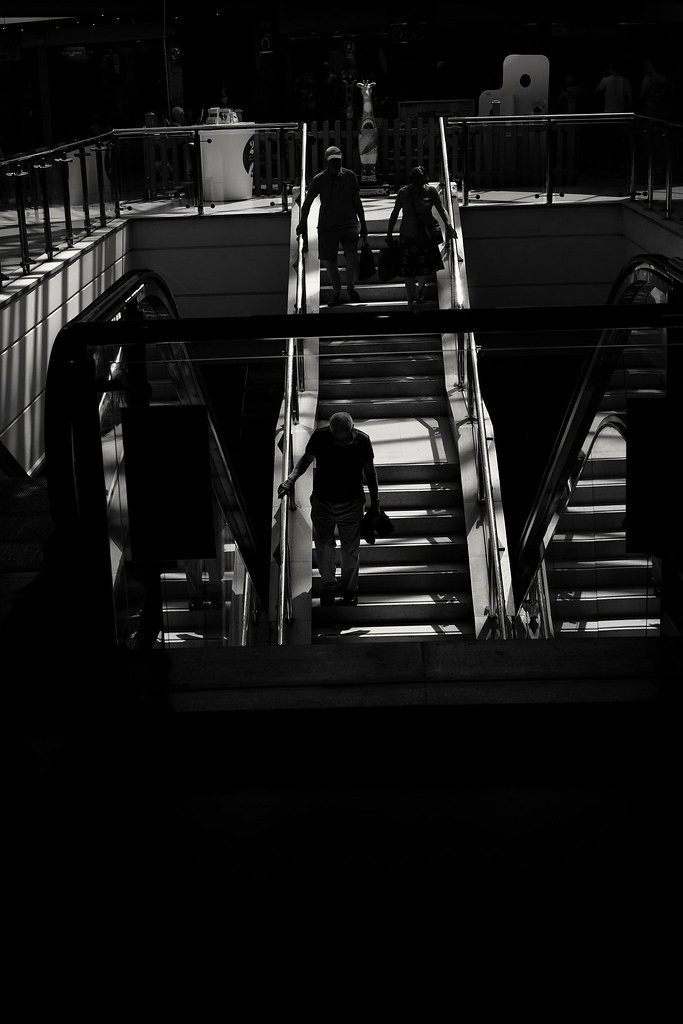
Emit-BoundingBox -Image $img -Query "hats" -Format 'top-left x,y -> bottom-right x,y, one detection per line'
325,146 -> 343,161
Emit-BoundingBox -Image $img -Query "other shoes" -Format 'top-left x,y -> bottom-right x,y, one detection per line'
188,599 -> 211,611
346,286 -> 361,302
320,597 -> 335,606
417,296 -> 425,304
342,594 -> 358,607
408,298 -> 417,311
327,296 -> 345,307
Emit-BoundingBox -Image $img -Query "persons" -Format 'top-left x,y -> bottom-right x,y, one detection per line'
638,59 -> 674,118
599,63 -> 632,112
385,167 -> 457,309
278,412 -> 378,607
170,107 -> 184,127
297,146 -> 366,307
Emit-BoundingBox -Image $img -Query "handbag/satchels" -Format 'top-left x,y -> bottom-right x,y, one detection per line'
418,218 -> 443,246
378,245 -> 399,275
360,239 -> 376,275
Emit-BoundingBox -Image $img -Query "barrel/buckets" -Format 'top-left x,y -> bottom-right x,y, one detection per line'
198,121 -> 255,201
